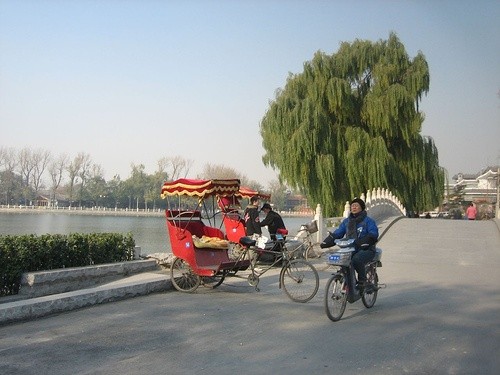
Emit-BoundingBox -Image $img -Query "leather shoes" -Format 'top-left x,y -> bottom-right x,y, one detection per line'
358,282 -> 366,288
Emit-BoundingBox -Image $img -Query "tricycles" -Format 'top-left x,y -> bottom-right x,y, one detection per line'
214,185 -> 333,271
160,177 -> 319,302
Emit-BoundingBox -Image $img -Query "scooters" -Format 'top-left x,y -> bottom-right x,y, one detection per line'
325,227 -> 387,322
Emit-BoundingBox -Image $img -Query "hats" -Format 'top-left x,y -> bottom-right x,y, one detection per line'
260,203 -> 273,210
350,198 -> 365,211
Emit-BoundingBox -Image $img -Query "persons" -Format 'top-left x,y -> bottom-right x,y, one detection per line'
408,213 -> 431,218
260,203 -> 286,267
466,204 -> 477,220
327,197 -> 379,289
244,196 -> 264,272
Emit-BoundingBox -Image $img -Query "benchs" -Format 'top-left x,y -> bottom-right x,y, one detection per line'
166,210 -> 224,249
